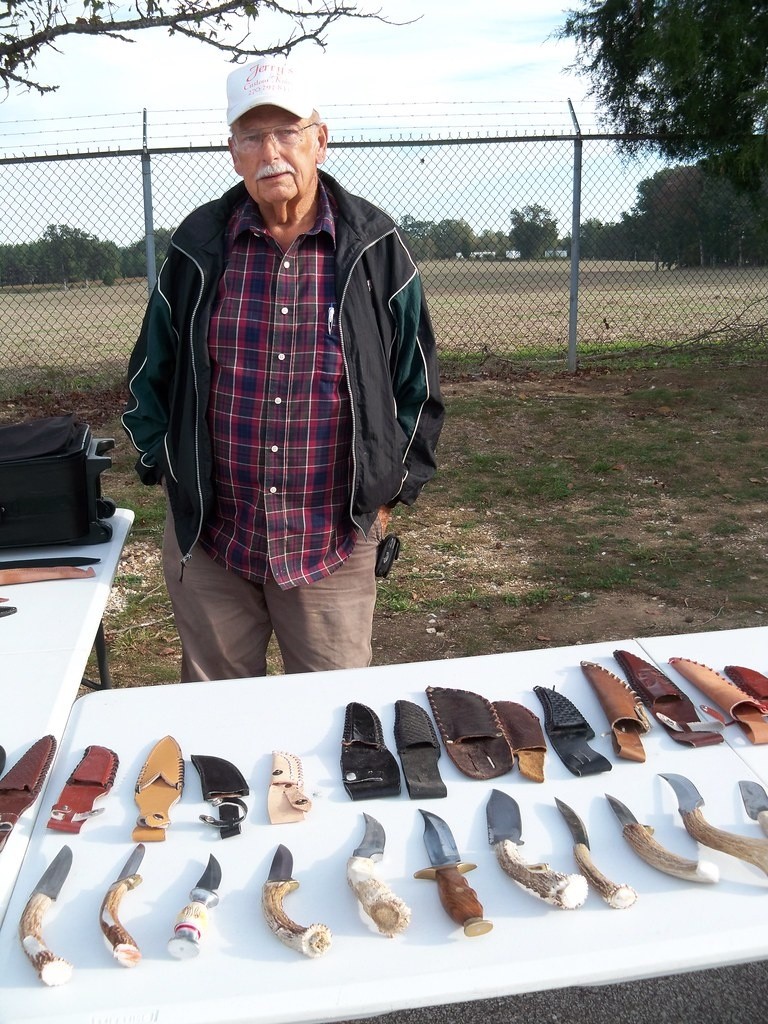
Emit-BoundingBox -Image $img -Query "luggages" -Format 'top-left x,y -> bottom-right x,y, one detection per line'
0,414 -> 116,550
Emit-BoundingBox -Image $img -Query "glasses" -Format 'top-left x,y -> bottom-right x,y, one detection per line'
233,122 -> 318,150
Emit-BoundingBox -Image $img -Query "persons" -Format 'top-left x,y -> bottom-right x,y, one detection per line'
121,58 -> 445,682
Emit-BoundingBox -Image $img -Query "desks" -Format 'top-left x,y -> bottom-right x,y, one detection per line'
0,620 -> 768,1024
0,506 -> 134,928
633,626 -> 768,794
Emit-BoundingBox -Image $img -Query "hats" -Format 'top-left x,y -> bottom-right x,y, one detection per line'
227,60 -> 317,125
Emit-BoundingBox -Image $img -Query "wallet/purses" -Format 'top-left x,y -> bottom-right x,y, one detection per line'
376,534 -> 399,576
